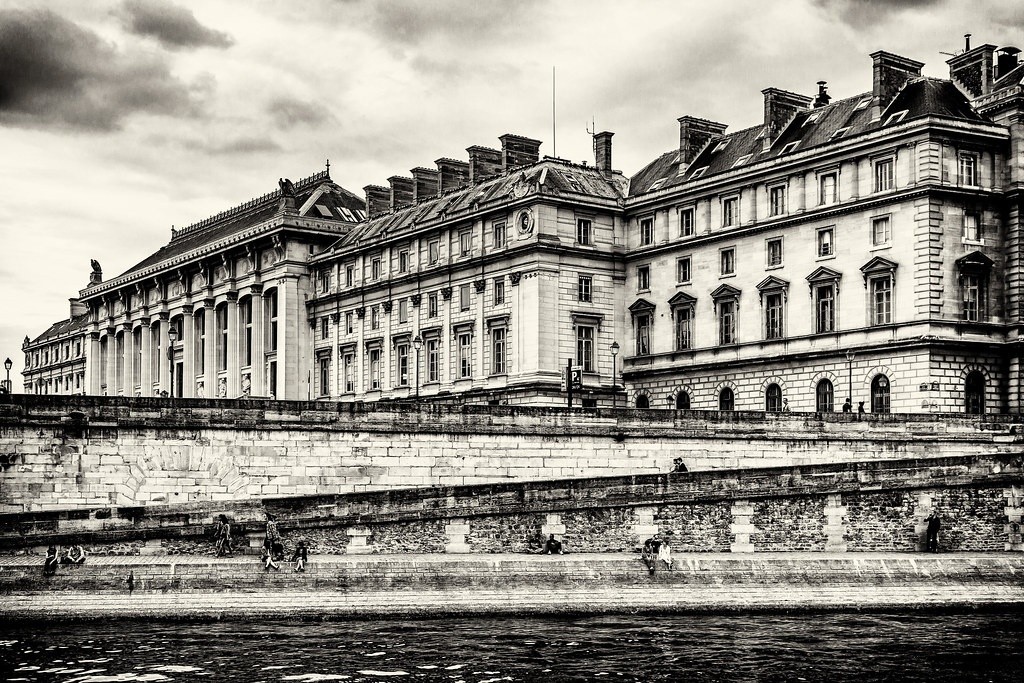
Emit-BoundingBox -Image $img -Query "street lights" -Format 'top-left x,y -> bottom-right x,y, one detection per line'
168,325 -> 180,399
610,341 -> 620,409
4,357 -> 13,394
845,349 -> 856,412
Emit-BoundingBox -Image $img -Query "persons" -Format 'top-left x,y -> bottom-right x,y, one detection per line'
659,540 -> 673,570
215,518 -> 234,558
652,535 -> 663,552
291,541 -> 308,572
134,373 -> 254,398
842,398 -> 852,412
527,534 -> 543,554
669,459 -> 679,473
923,511 -> 940,553
41,543 -> 58,578
260,540 -> 280,571
213,514 -> 226,556
782,398 -> 791,412
677,458 -> 688,472
641,539 -> 656,573
858,402 -> 865,413
66,542 -> 86,565
266,515 -> 280,557
545,534 -> 564,555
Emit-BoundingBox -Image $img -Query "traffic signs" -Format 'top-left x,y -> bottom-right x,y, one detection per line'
571,366 -> 583,390
